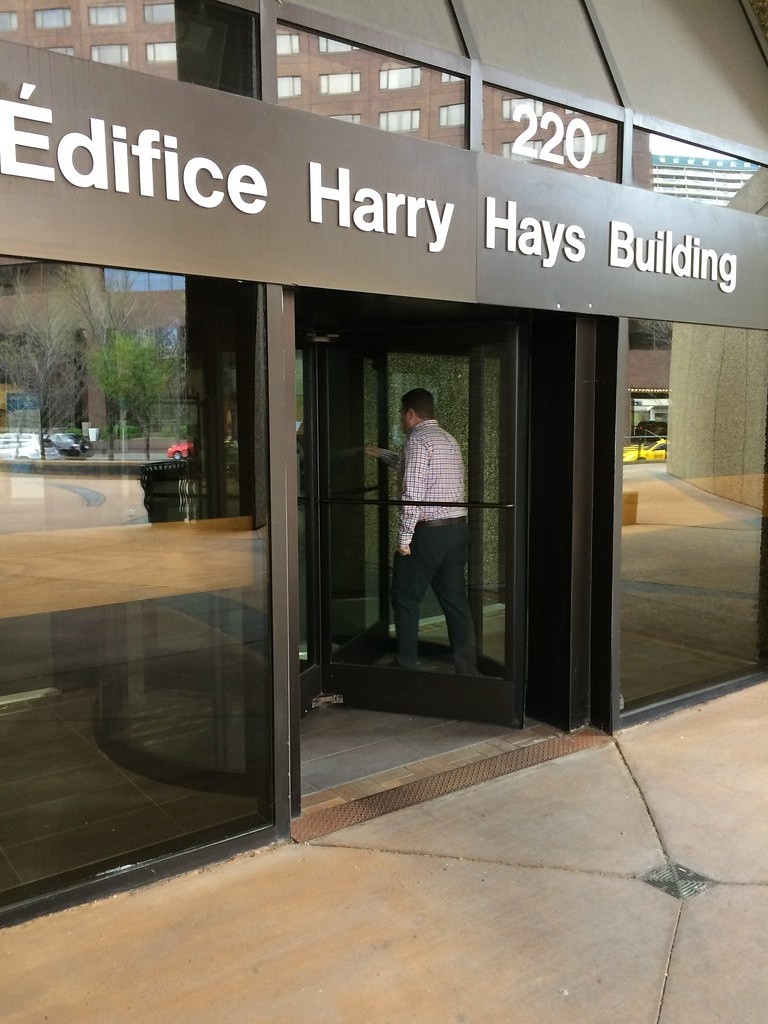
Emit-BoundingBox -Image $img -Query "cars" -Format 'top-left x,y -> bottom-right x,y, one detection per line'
0,431 -> 94,461
622,420 -> 667,462
167,441 -> 194,460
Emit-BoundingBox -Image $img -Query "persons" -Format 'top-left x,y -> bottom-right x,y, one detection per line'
382,388 -> 478,678
